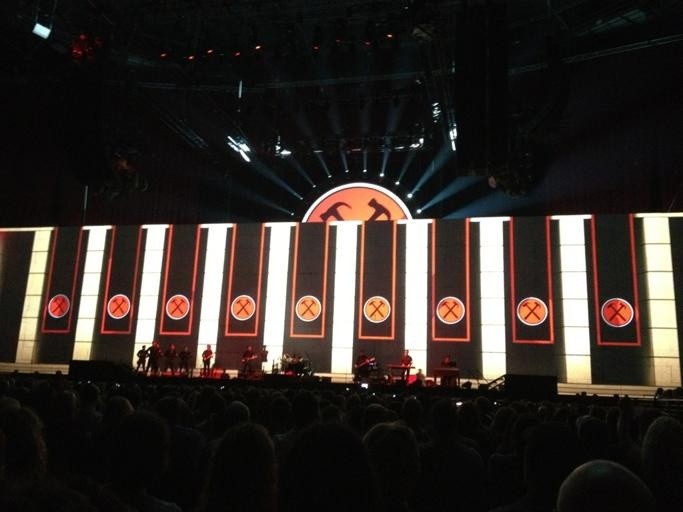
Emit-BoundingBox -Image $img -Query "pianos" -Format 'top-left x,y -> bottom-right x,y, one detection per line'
432,366 -> 459,387
387,364 -> 414,368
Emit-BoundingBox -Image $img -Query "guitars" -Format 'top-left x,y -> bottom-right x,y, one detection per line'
203,352 -> 214,361
354,357 -> 375,368
241,355 -> 257,364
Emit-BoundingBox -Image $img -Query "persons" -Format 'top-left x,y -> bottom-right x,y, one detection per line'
2,377 -> 682,511
202,345 -> 213,376
136,343 -> 194,376
241,344 -> 255,374
440,355 -> 456,385
353,350 -> 369,382
400,350 -> 412,382
